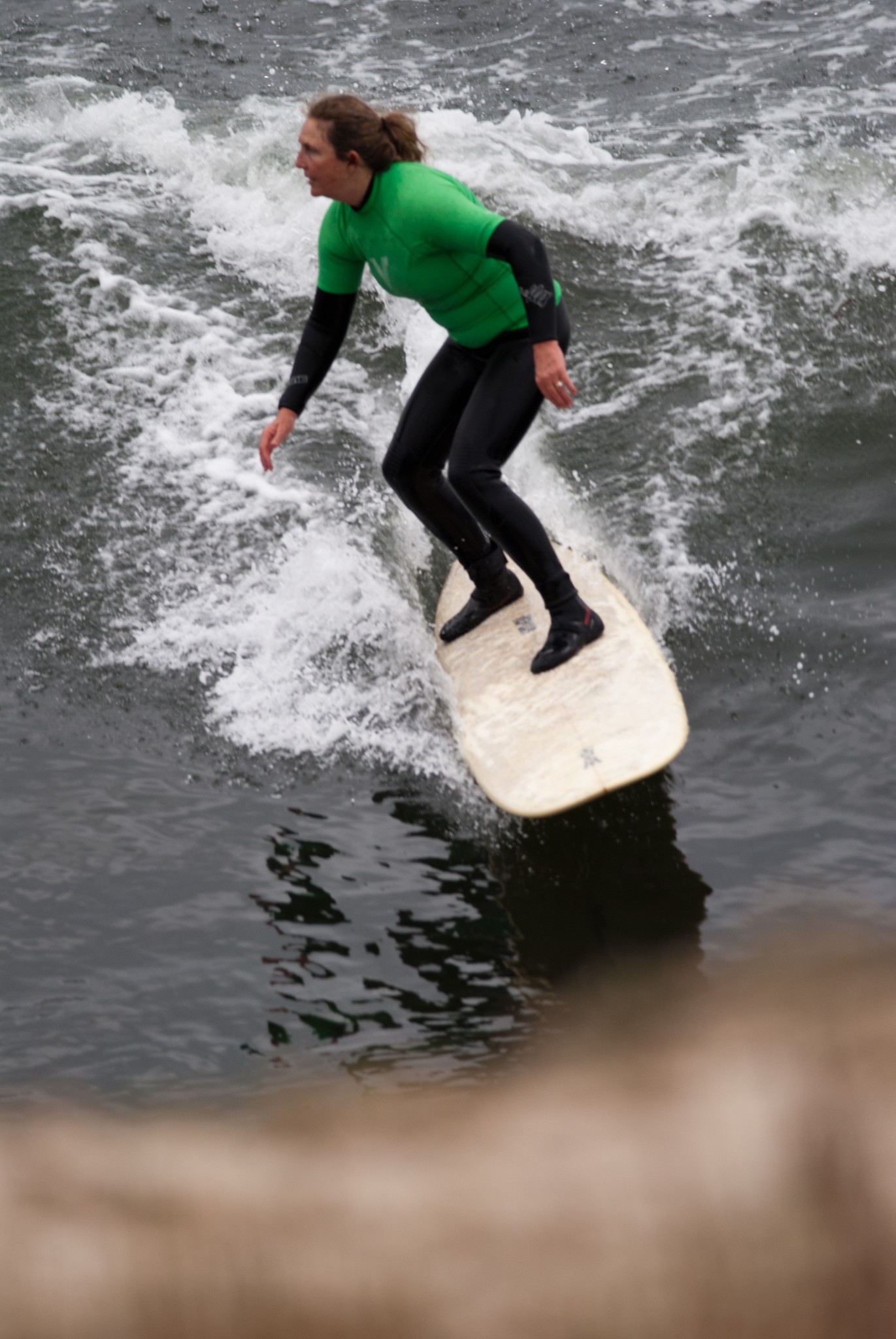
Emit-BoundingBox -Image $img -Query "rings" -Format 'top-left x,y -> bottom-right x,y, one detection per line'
555,380 -> 563,388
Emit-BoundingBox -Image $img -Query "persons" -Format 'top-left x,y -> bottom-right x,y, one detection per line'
259,96 -> 604,672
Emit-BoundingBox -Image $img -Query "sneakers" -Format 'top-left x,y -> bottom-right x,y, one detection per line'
530,595 -> 603,674
439,571 -> 523,642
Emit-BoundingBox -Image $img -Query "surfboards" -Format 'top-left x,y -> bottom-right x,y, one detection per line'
433,538 -> 689,818
